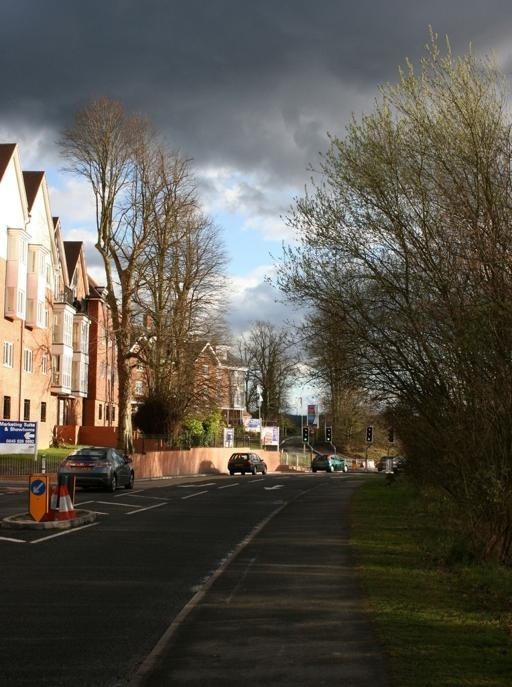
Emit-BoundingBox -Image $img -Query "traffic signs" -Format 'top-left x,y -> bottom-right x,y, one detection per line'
0,420 -> 37,445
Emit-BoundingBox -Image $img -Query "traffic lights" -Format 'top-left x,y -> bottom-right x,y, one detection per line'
325,427 -> 332,442
387,428 -> 395,443
366,426 -> 373,442
302,427 -> 309,443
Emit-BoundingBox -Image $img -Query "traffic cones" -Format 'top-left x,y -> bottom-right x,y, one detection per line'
57,483 -> 78,520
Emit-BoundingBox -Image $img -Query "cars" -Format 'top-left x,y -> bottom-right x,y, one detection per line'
228,452 -> 266,475
57,446 -> 135,491
311,454 -> 348,472
376,456 -> 401,471
